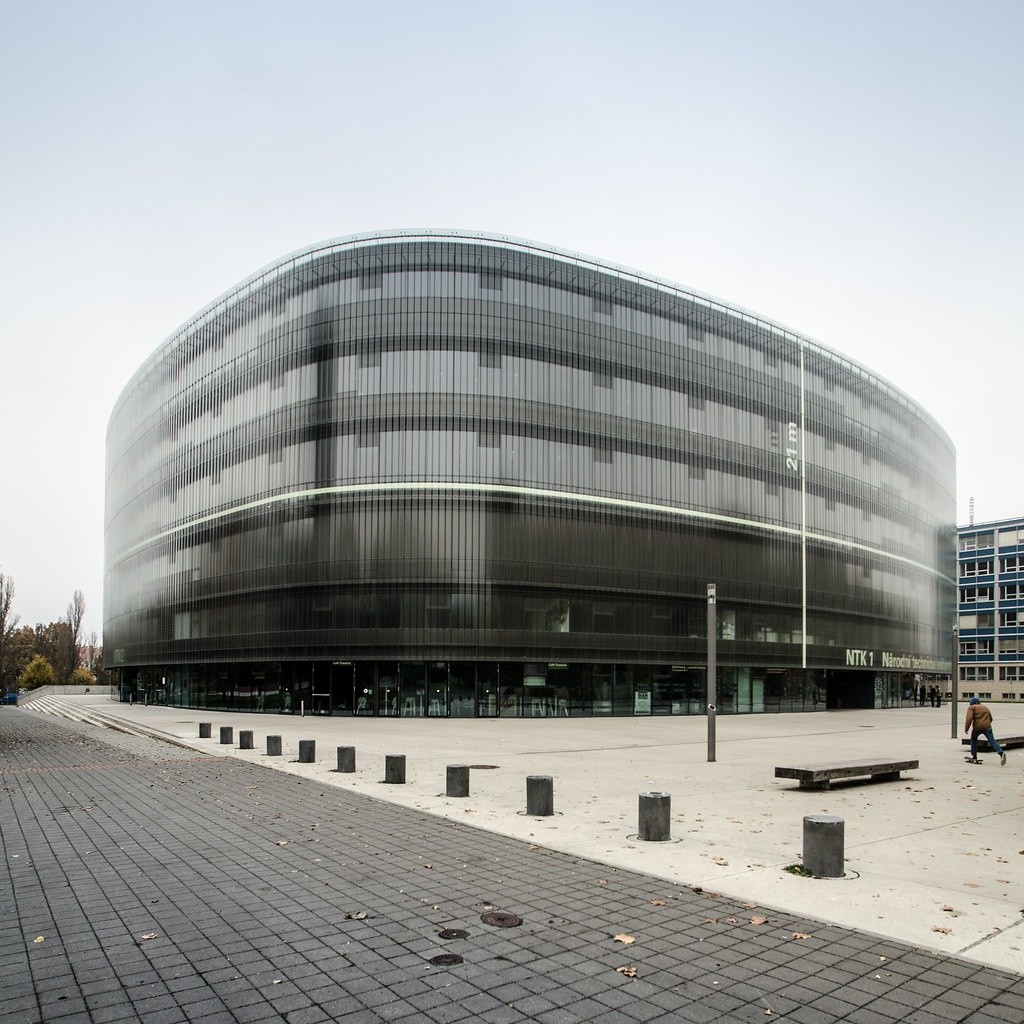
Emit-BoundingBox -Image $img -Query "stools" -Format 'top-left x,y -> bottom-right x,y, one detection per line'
429,699 -> 441,715
530,699 -> 543,716
403,697 -> 417,717
557,700 -> 568,716
542,699 -> 554,716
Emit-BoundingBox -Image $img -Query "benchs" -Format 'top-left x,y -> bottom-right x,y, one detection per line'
962,733 -> 1024,752
775,757 -> 919,791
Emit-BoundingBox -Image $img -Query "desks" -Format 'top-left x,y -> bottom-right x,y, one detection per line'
486,694 -> 517,716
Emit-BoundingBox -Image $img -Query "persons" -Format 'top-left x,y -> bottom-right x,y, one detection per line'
935,685 -> 941,707
965,698 -> 1006,765
929,685 -> 936,707
920,685 -> 926,706
881,673 -> 919,708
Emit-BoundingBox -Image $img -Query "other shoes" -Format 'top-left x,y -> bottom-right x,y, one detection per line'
970,754 -> 977,760
1000,753 -> 1007,766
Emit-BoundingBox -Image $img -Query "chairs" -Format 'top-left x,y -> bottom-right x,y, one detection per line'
392,697 -> 399,715
356,697 -> 366,714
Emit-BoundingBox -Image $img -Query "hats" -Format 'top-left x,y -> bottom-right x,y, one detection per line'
970,697 -> 981,705
